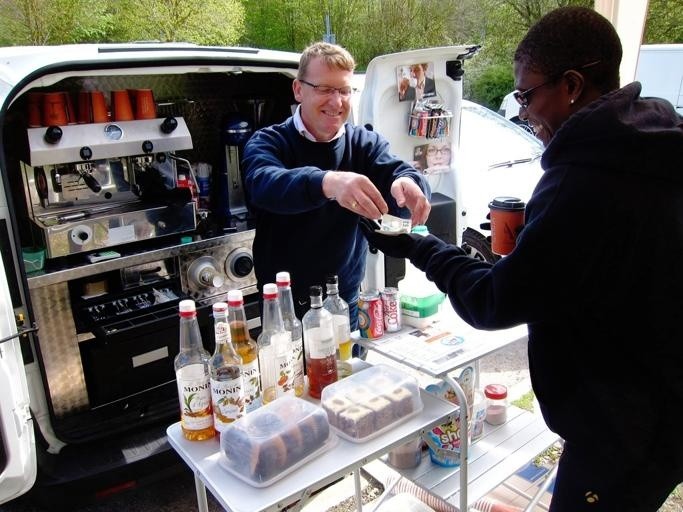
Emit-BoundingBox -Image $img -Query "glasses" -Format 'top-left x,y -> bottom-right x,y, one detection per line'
514,61 -> 600,109
299,80 -> 358,98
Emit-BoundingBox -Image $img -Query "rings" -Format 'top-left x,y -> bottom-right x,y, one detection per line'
352,201 -> 358,210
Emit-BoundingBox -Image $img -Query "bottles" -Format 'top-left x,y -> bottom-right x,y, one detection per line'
177,174 -> 187,187
303,285 -> 337,399
173,270 -> 301,440
326,272 -> 351,363
195,164 -> 214,202
483,384 -> 508,425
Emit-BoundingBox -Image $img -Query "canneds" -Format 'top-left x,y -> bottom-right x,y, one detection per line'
357,295 -> 384,338
379,287 -> 401,333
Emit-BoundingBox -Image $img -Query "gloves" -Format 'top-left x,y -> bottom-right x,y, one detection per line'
357,216 -> 424,259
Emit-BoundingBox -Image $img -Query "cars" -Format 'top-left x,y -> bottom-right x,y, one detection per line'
350,73 -> 550,269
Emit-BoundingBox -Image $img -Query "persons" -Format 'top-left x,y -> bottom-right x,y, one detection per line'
398,64 -> 434,100
241,41 -> 431,357
418,141 -> 450,174
359,7 -> 683,512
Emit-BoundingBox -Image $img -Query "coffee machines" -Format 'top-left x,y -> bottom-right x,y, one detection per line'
209,125 -> 255,219
11,114 -> 197,260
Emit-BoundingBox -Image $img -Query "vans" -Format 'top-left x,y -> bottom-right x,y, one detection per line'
0,43 -> 483,512
495,43 -> 682,141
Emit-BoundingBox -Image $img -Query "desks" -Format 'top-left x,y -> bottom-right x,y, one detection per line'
349,308 -> 561,512
165,356 -> 459,512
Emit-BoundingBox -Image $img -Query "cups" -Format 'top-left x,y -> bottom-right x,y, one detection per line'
194,263 -> 225,289
487,196 -> 525,254
29,87 -> 177,127
